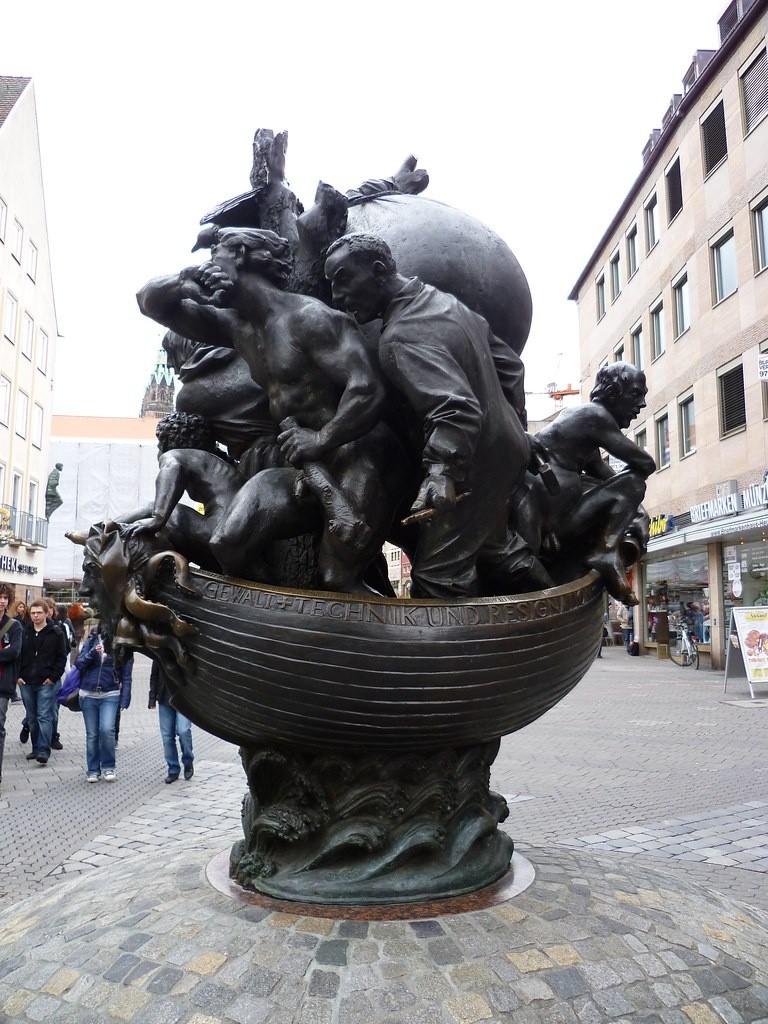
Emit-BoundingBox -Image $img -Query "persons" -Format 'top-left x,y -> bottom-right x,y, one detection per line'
597,644 -> 602,658
46,462 -> 63,521
136,227 -> 657,607
0,583 -> 194,784
616,603 -> 634,653
686,601 -> 704,643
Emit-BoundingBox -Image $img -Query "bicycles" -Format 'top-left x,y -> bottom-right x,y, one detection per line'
667,614 -> 700,670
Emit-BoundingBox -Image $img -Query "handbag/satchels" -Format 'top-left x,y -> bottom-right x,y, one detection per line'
56,667 -> 82,711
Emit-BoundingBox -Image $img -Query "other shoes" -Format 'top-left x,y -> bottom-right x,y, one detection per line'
52,733 -> 63,750
165,775 -> 178,783
27,753 -> 37,759
184,766 -> 194,779
20,724 -> 29,743
37,754 -> 48,763
86,773 -> 99,782
102,769 -> 116,781
114,741 -> 119,751
597,655 -> 603,658
